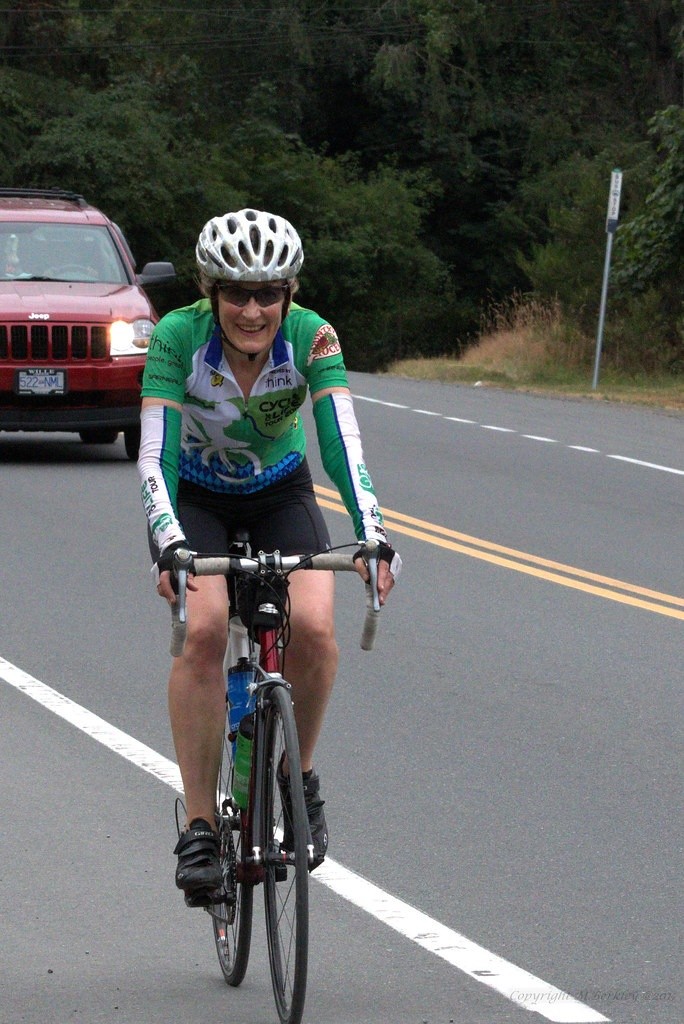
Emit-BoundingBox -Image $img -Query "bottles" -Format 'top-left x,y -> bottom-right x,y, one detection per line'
227,655 -> 258,759
232,713 -> 256,808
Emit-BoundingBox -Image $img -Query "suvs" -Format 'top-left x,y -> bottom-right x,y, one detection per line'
0,185 -> 179,462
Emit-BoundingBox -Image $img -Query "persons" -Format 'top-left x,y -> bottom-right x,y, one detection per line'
136,204 -> 406,908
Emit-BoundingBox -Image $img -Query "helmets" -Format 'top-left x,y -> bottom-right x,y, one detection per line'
193,207 -> 306,282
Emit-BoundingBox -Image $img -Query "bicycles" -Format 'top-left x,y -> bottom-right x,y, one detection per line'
166,524 -> 383,1024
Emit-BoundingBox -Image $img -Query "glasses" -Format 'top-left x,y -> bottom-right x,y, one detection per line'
216,286 -> 290,310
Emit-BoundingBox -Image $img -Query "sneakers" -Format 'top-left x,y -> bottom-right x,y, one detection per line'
175,818 -> 225,892
276,748 -> 328,873
